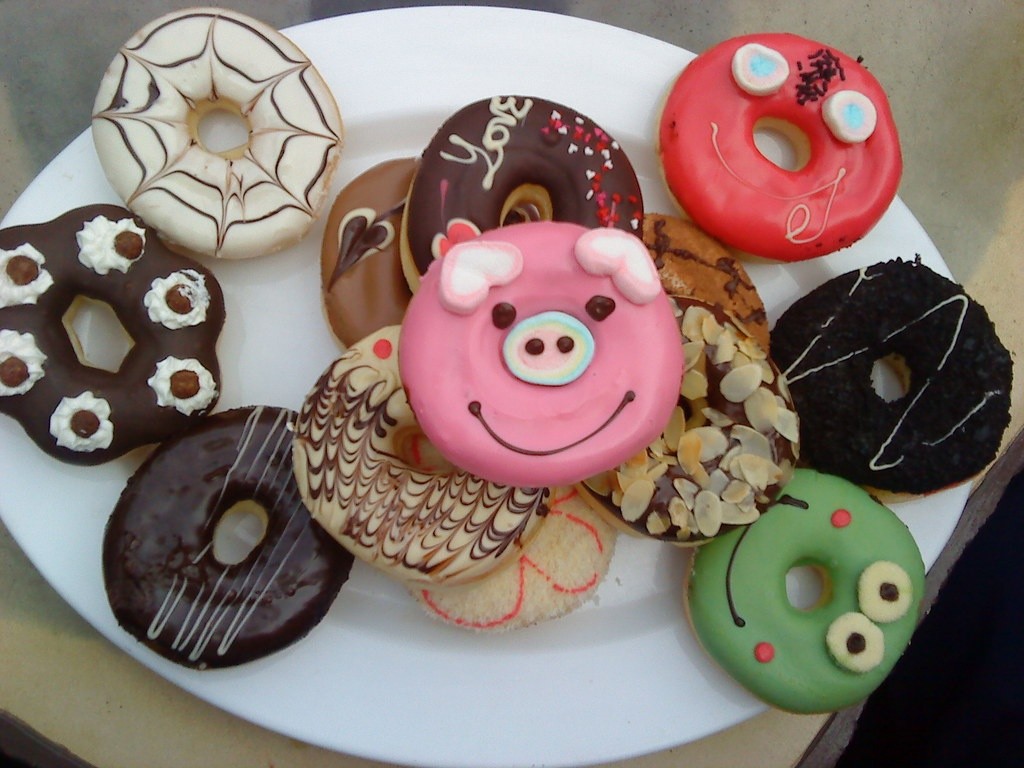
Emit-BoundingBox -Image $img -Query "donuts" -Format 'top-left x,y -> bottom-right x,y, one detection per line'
1,5 -> 1018,718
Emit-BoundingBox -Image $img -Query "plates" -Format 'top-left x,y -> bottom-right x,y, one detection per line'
0,5 -> 976,767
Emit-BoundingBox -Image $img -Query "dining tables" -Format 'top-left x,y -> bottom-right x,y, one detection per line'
2,1 -> 1023,768
